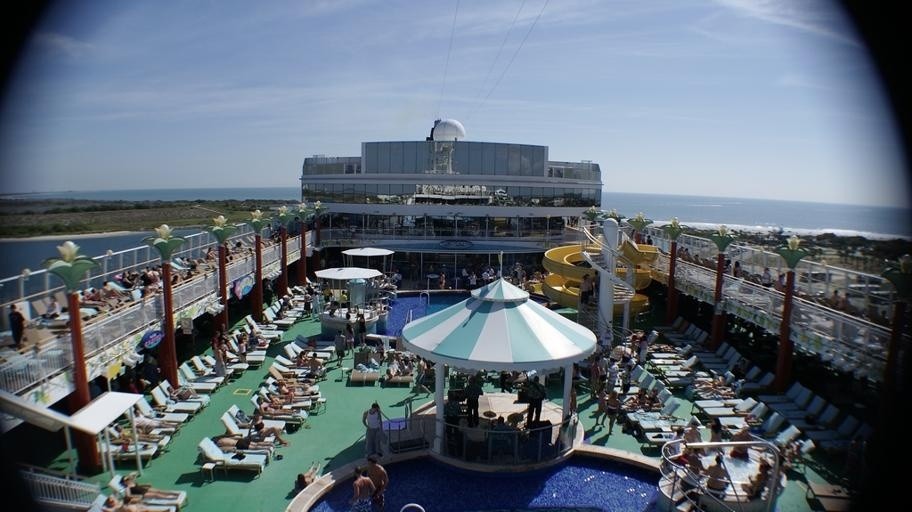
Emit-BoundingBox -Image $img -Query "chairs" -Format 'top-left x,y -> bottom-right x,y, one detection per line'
348,347 -> 588,387
94,276 -> 337,481
593,316 -> 881,491
448,421 -> 553,459
88,474 -> 188,512
632,235 -> 899,345
2,235 -> 285,378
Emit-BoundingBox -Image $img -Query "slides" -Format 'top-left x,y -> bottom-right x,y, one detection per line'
531,244 -> 659,316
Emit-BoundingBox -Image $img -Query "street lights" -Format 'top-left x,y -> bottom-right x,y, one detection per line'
880,254 -> 912,393
139,224 -> 190,392
243,208 -> 274,324
295,201 -> 312,287
310,198 -> 329,280
202,213 -> 239,338
707,225 -> 734,356
661,218 -> 689,325
277,204 -> 291,299
584,206 -> 653,243
775,234 -> 808,389
41,240 -> 103,476
